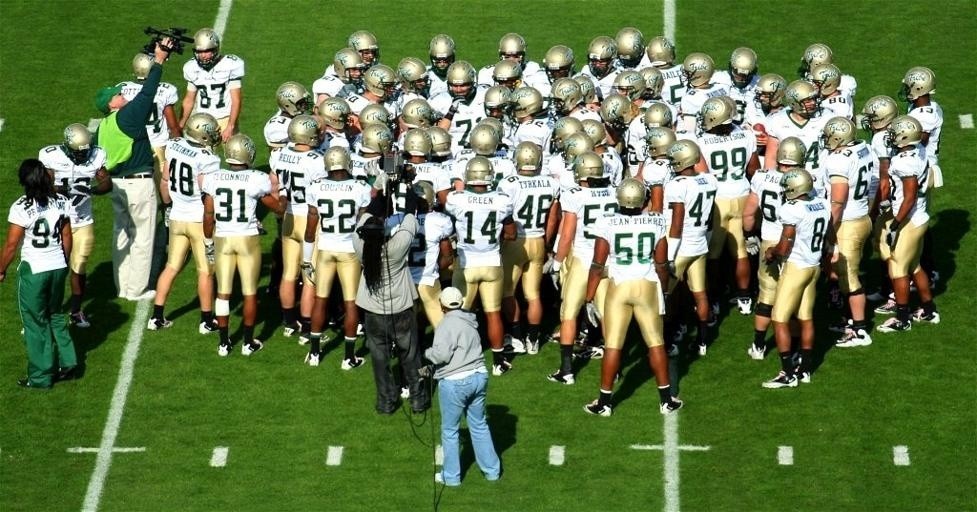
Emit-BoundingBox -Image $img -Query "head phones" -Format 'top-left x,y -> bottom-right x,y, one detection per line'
356,225 -> 385,239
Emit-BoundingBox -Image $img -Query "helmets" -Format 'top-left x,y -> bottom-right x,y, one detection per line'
644,103 -> 672,131
224,133 -> 257,169
483,85 -> 513,121
587,36 -> 618,79
700,95 -> 738,132
62,122 -> 92,152
191,27 -> 220,61
639,66 -> 664,100
614,177 -> 647,211
563,132 -> 594,168
513,141 -> 541,176
470,125 -> 499,156
131,52 -> 153,81
822,94 -> 923,152
445,58 -> 478,99
666,139 -> 700,173
645,128 -> 675,158
552,116 -> 585,152
411,179 -> 435,210
901,65 -> 938,104
464,157 -> 494,186
775,135 -> 814,201
600,95 -> 633,125
729,43 -> 841,120
551,78 -> 584,116
573,152 -> 604,183
274,29 -> 456,176
182,112 -> 222,147
492,60 -> 522,87
615,27 -> 646,67
647,35 -> 676,63
574,76 -> 595,104
581,119 -> 607,148
477,117 -> 503,141
612,72 -> 646,102
542,44 -> 576,85
682,52 -> 715,87
510,86 -> 543,118
497,32 -> 528,61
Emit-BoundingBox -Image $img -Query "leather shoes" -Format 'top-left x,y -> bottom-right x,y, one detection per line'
54,362 -> 79,381
16,376 -> 54,390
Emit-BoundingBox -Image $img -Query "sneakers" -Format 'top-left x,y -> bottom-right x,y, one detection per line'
659,396 -> 684,416
582,398 -> 613,417
434,471 -> 447,485
736,296 -> 753,316
546,368 -> 576,386
491,328 -> 606,362
761,370 -> 799,389
127,288 -> 158,302
746,341 -> 768,361
68,310 -> 92,329
217,337 -> 233,357
198,319 -> 220,335
146,315 -> 174,331
282,312 -> 366,372
829,268 -> 941,349
241,337 -> 264,356
666,301 -> 721,358
793,369 -> 812,384
491,357 -> 513,376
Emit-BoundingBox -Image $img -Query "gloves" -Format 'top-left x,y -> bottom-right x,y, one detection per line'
300,260 -> 317,287
742,228 -> 762,257
542,251 -> 561,291
584,298 -> 601,328
886,220 -> 901,252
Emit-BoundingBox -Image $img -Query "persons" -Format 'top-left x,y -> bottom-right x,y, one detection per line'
264,27 -> 943,417
423,287 -> 501,487
201,133 -> 281,356
0,28 -> 244,388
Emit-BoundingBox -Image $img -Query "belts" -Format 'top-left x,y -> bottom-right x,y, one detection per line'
119,173 -> 153,179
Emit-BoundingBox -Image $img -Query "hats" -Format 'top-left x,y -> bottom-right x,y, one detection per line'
437,285 -> 465,311
94,83 -> 123,114
